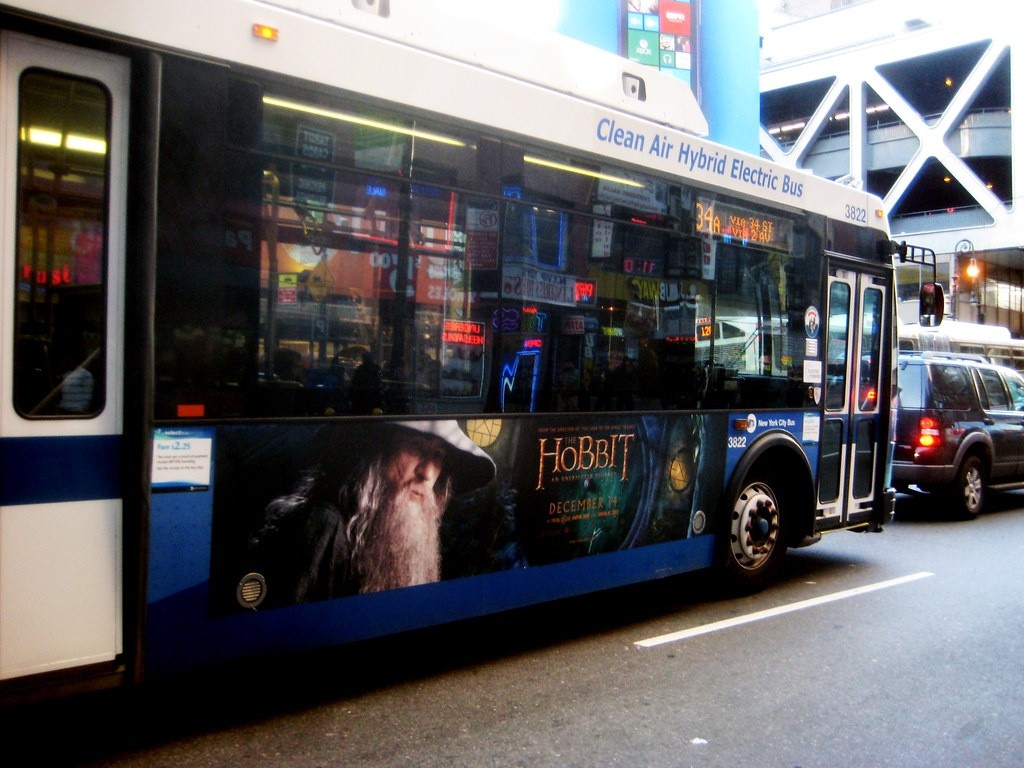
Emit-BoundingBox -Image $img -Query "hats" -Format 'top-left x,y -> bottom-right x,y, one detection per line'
390,420 -> 498,504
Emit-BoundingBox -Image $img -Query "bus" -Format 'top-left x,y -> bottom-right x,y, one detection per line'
1,1 -> 946,709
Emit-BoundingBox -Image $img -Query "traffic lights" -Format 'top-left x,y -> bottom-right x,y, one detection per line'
918,281 -> 943,330
961,253 -> 982,284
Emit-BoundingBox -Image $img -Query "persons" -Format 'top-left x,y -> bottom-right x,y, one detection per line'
248,419 -> 498,608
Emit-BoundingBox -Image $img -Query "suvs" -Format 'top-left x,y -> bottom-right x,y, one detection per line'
841,349 -> 1023,522
